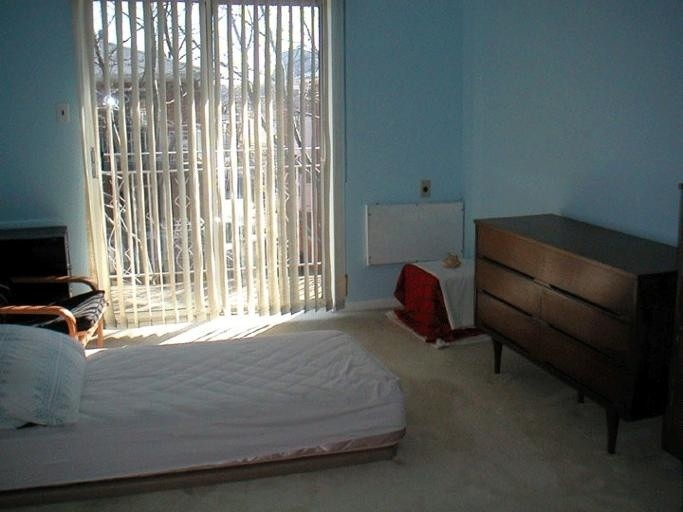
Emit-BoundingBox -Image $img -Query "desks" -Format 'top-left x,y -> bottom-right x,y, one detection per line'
0,223 -> 70,302
397,258 -> 489,347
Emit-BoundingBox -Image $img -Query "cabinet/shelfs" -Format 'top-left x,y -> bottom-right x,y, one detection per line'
469,213 -> 682,454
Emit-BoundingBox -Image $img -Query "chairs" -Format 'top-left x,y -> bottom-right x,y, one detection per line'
0,270 -> 110,352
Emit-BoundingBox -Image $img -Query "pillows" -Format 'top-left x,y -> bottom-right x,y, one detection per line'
0,320 -> 86,432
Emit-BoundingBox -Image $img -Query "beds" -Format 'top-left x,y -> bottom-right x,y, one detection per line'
0,316 -> 408,507
